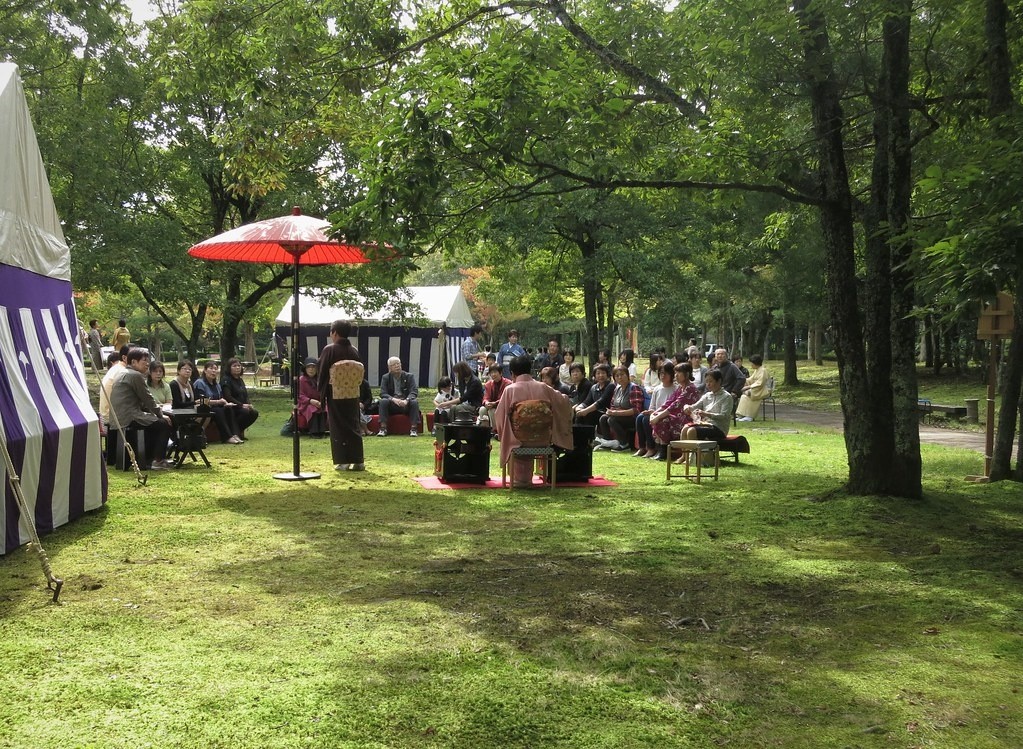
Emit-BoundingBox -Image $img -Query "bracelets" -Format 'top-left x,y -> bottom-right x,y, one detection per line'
391,397 -> 393,401
309,399 -> 311,404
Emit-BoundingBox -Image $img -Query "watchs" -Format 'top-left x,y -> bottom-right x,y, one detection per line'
406,398 -> 409,401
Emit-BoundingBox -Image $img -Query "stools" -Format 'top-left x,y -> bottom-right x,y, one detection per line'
666,440 -> 720,485
103,423 -> 149,473
502,448 -> 557,493
259,380 -> 274,387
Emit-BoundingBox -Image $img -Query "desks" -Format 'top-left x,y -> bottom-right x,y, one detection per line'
435,423 -> 596,484
162,408 -> 215,470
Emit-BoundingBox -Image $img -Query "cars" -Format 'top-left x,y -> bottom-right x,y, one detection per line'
699,344 -> 729,358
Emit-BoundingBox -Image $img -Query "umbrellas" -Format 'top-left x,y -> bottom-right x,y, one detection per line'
188,205 -> 402,476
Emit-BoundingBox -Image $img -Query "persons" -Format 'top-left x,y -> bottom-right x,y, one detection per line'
376,356 -> 419,437
299,357 -> 328,439
317,320 -> 366,471
359,378 -> 376,435
90,318 -> 258,471
433,326 -> 770,489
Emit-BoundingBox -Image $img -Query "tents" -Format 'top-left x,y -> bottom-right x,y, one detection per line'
276,286 -> 476,389
1,63 -> 108,554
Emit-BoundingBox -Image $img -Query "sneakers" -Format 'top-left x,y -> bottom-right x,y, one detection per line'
151,460 -> 173,470
410,427 -> 418,437
377,427 -> 388,437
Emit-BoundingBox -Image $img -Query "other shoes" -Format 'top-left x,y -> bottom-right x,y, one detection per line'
643,451 -> 657,457
335,463 -> 351,470
359,427 -> 374,436
632,449 -> 647,456
610,444 -> 631,452
349,463 -> 365,471
310,432 -> 328,439
649,452 -> 667,461
490,431 -> 498,440
673,456 -> 686,464
598,445 -> 611,450
222,434 -> 244,444
735,414 -> 754,422
513,478 -> 533,487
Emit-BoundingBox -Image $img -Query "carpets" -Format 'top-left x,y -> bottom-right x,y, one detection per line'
411,474 -> 619,490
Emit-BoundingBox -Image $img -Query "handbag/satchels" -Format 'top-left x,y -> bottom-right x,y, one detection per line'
280,415 -> 295,435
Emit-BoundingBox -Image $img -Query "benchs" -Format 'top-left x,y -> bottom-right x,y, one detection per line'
426,413 -> 449,432
918,404 -> 967,420
365,414 -> 423,435
609,427 -> 750,464
291,412 -> 309,435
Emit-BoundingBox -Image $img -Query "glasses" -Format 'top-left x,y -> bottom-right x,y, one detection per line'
181,367 -> 193,372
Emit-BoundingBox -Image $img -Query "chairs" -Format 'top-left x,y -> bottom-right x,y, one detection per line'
762,377 -> 776,422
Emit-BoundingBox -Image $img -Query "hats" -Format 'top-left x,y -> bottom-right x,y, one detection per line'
305,357 -> 317,367
